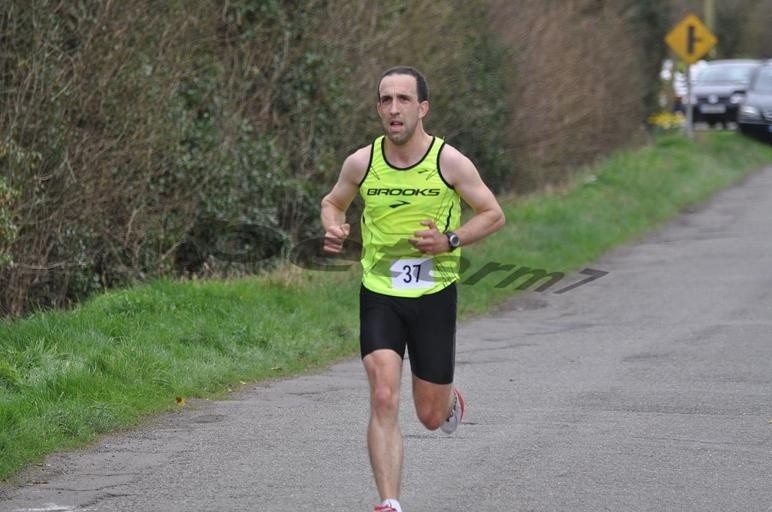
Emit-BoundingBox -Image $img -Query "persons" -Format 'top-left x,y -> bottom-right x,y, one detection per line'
319,66 -> 508,512
661,59 -> 687,117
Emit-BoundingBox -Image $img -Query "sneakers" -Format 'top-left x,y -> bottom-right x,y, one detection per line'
439,384 -> 464,434
373,501 -> 402,512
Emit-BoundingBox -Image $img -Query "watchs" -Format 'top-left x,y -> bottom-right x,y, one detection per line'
443,232 -> 462,255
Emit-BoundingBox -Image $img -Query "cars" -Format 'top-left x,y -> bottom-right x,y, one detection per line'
658,58 -> 771,142
